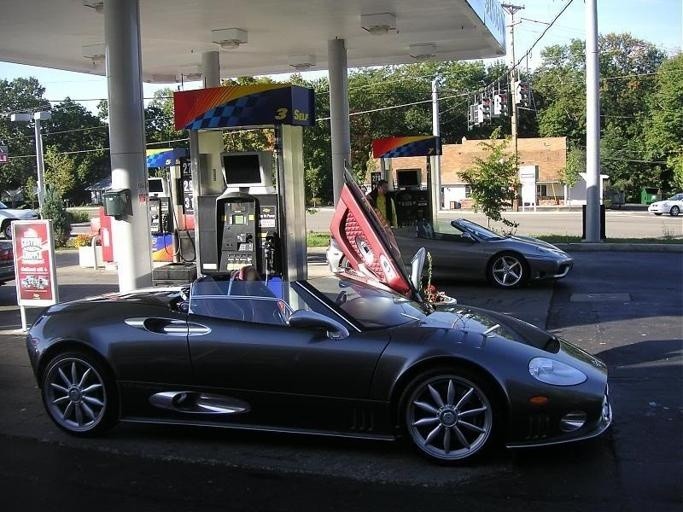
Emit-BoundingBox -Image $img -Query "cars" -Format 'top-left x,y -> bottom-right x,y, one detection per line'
647,193 -> 682,218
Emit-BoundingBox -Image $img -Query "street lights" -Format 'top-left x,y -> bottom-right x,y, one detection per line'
9,110 -> 52,208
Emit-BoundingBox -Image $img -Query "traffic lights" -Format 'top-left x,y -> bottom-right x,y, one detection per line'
517,80 -> 530,108
480,97 -> 492,126
498,90 -> 511,117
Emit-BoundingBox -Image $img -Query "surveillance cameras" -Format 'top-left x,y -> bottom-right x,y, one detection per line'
11,114 -> 30,121
34,111 -> 50,120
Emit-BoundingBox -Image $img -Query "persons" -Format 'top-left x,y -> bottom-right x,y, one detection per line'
367,179 -> 397,230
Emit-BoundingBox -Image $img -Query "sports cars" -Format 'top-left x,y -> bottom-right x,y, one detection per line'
24,270 -> 611,464
327,216 -> 573,290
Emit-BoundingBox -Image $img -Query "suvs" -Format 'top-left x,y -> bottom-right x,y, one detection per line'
0,201 -> 38,284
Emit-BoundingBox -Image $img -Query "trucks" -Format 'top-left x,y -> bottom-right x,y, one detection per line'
148,176 -> 167,224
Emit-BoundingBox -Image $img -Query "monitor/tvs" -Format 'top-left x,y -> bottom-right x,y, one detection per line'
220,152 -> 272,193
397,169 -> 421,191
148,178 -> 165,196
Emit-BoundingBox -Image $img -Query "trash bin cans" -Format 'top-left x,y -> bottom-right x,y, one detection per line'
582,205 -> 606,239
641,187 -> 662,205
608,190 -> 625,204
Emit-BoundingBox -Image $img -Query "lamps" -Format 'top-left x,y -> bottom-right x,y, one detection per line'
286,54 -> 318,72
359,11 -> 398,36
406,42 -> 436,61
178,65 -> 203,82
209,24 -> 249,52
79,42 -> 107,67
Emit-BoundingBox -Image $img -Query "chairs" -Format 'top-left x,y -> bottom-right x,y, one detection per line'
188,275 -> 243,323
